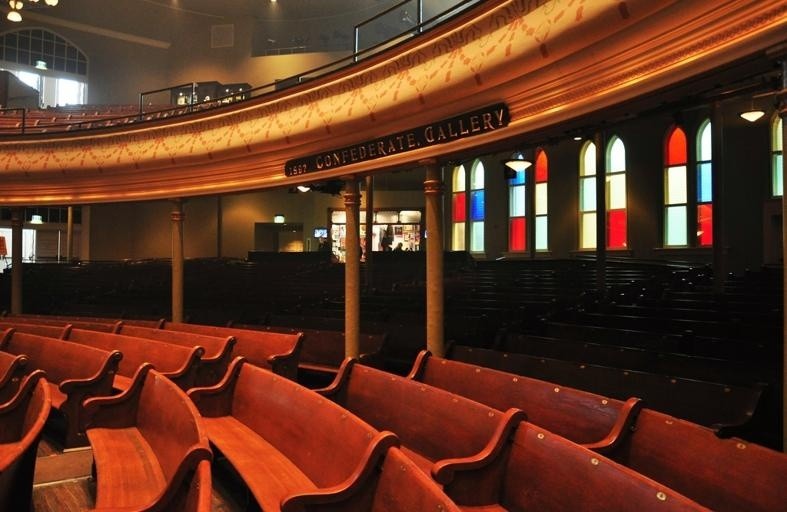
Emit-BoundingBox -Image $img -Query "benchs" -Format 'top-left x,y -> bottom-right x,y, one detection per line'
0,102 -> 227,135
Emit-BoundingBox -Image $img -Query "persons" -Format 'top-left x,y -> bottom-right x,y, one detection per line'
393,243 -> 404,250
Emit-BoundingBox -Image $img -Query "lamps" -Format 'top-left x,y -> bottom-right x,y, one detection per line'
505,146 -> 532,172
739,99 -> 766,122
